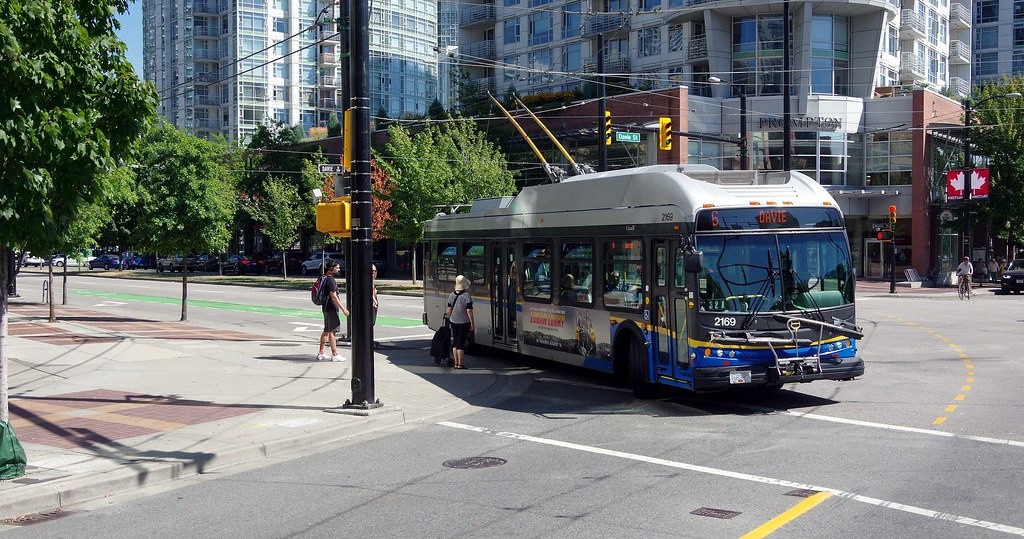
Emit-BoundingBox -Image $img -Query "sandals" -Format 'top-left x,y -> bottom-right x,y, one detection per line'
457,364 -> 468,369
454,364 -> 458,368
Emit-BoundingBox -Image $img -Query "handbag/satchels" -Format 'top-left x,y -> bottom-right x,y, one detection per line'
983,266 -> 987,275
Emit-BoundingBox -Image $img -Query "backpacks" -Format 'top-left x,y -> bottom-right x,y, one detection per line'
311,275 -> 337,306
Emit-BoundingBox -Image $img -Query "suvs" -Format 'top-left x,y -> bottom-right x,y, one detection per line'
121,250 -> 152,270
157,250 -> 196,272
301,252 -> 344,276
265,252 -> 308,275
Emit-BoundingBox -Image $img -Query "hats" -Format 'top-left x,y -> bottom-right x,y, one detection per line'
454,275 -> 471,291
1002,259 -> 1007,262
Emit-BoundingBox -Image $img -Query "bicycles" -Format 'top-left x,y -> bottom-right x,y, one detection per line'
956,274 -> 973,300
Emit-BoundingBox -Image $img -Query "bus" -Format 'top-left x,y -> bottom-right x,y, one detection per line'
421,161 -> 864,394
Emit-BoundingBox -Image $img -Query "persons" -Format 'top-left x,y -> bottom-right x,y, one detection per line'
1000,259 -> 1008,275
560,274 -> 578,297
372,264 -> 378,326
956,256 -> 973,300
448,275 -> 475,369
975,259 -> 987,286
508,260 -> 516,321
988,258 -> 1000,284
316,263 -> 350,361
608,270 -> 629,292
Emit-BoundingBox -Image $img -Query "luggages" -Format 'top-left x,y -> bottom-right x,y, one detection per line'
430,313 -> 454,367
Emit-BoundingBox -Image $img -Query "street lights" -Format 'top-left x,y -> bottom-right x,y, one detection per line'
963,93 -> 1020,256
708,77 -> 747,170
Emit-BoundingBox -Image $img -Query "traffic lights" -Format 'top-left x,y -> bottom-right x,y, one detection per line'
889,204 -> 897,224
660,117 -> 673,151
877,231 -> 890,240
606,112 -> 612,145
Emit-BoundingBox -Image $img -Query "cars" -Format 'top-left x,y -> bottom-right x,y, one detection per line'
219,254 -> 265,276
196,253 -> 222,272
89,254 -> 126,270
20,251 -> 45,267
373,252 -> 406,275
52,254 -> 90,267
1001,258 -> 1024,294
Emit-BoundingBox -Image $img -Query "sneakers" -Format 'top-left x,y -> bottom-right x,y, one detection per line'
317,353 -> 330,360
331,353 -> 346,362
